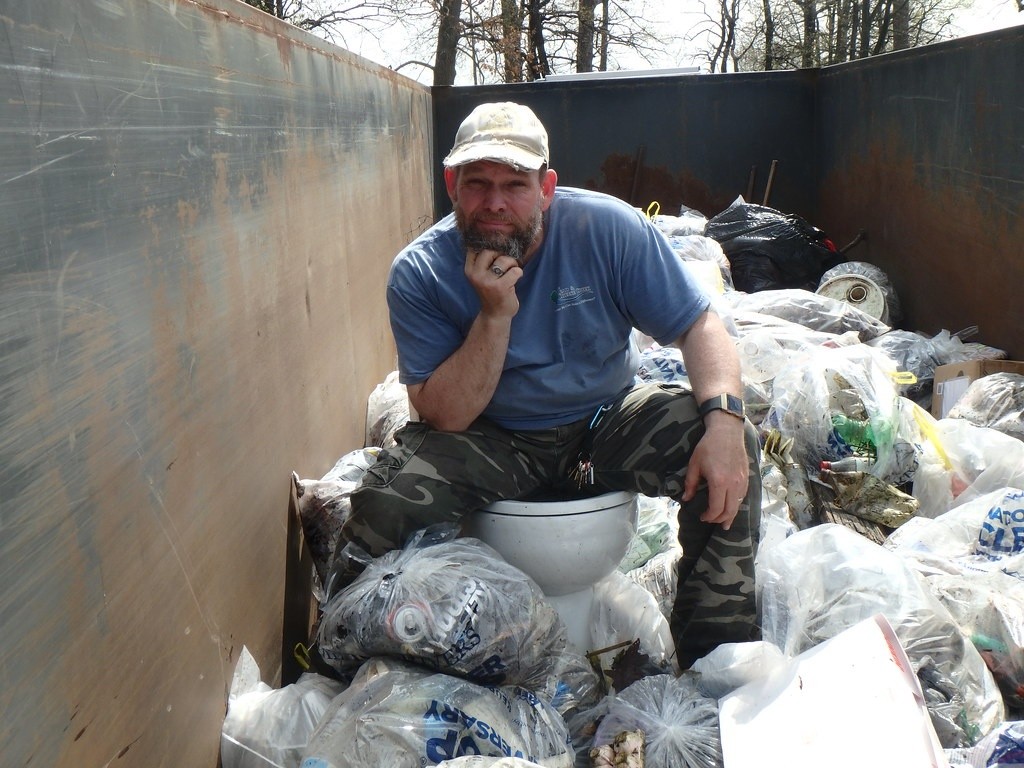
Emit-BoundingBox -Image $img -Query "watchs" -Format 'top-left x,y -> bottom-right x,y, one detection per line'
699,392 -> 746,422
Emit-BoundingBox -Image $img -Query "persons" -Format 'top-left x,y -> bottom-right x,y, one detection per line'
310,101 -> 761,673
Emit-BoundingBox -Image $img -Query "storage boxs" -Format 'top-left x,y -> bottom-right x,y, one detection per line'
718,611 -> 950,768
931,359 -> 1024,420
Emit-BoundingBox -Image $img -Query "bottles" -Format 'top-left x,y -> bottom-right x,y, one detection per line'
821,415 -> 919,529
759,449 -> 788,500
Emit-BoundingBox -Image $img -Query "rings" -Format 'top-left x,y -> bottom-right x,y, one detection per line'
490,265 -> 504,277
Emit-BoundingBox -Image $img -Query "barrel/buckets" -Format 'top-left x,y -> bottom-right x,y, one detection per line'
814,274 -> 895,332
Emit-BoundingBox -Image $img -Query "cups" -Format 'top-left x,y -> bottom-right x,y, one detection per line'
782,463 -> 819,529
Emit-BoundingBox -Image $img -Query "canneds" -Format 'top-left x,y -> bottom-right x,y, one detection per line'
387,602 -> 425,643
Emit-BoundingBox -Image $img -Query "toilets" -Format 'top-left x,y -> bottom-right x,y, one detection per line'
408,393 -> 640,663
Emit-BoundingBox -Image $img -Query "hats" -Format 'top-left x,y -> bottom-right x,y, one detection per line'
443,101 -> 549,170
